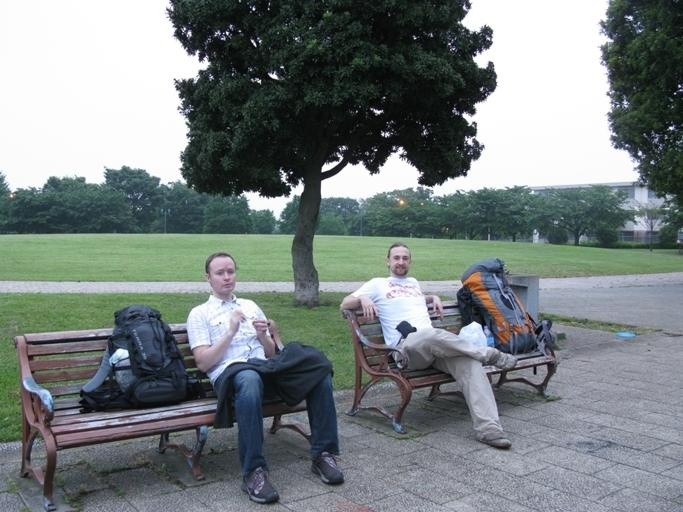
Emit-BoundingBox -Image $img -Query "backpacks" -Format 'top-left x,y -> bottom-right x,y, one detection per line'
80,301 -> 191,417
455,257 -> 558,361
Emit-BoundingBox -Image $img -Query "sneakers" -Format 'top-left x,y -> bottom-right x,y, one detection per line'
478,431 -> 513,449
491,348 -> 519,372
308,450 -> 345,486
238,465 -> 281,504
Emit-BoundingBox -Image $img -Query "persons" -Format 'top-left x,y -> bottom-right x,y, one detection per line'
340,243 -> 519,448
186,251 -> 346,506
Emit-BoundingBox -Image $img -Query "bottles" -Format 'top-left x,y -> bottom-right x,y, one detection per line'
483,326 -> 494,347
109,349 -> 133,390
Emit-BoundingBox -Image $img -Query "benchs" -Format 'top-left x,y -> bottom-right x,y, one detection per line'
343,300 -> 561,434
12,323 -> 312,512
